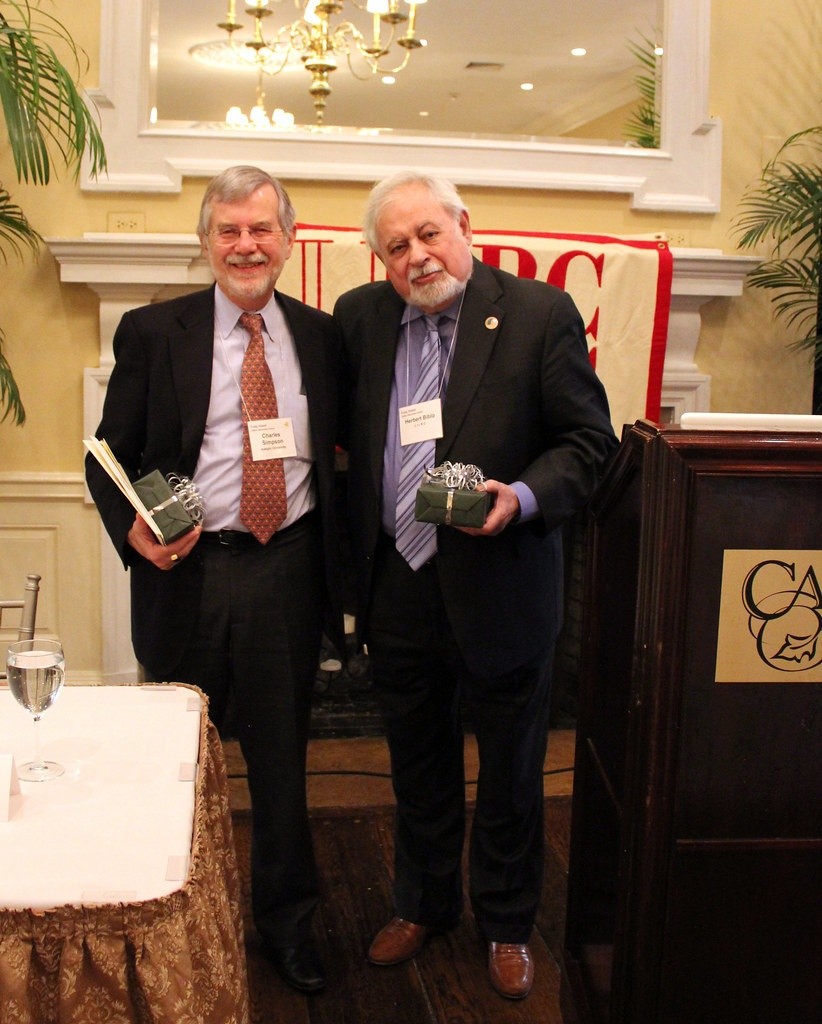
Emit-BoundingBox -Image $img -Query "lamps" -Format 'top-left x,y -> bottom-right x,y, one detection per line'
207,0 -> 422,125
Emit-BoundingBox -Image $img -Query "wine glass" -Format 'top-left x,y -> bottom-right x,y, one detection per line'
6,640 -> 68,782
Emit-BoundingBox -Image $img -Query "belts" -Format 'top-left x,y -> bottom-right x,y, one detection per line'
197,510 -> 316,547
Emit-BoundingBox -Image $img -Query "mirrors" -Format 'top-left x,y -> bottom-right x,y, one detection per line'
76,2 -> 724,213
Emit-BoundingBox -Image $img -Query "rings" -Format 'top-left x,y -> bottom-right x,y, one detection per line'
170,554 -> 178,560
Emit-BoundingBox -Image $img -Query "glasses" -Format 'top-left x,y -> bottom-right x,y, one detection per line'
202,222 -> 286,247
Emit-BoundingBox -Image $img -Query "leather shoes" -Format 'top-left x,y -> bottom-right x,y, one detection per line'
484,939 -> 534,1000
261,932 -> 327,992
368,914 -> 422,966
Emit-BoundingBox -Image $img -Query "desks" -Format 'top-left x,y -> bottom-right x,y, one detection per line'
2,677 -> 252,1024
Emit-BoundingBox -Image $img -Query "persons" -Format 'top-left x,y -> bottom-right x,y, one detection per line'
84,162 -> 341,991
326,167 -> 621,1000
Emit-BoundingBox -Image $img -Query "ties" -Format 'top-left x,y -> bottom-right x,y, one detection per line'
394,311 -> 444,571
240,313 -> 287,545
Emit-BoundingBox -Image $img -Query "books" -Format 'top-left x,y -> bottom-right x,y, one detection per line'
83,435 -> 167,547
680,412 -> 822,432
